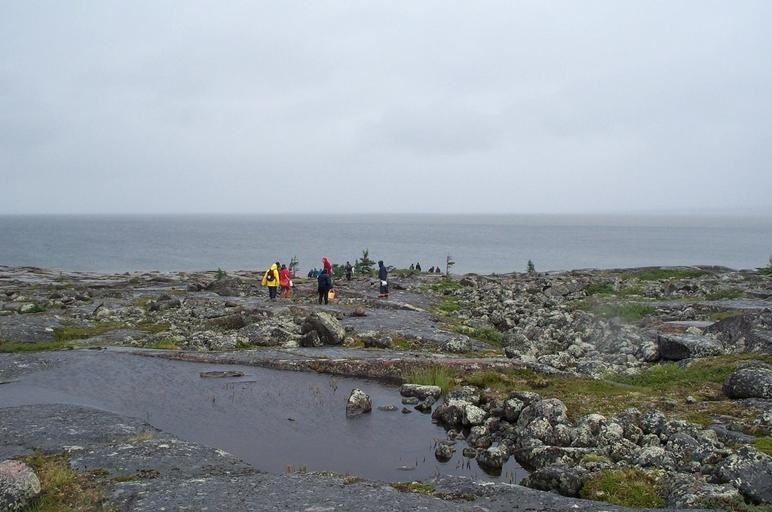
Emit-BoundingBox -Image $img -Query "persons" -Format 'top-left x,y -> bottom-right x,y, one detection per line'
345,262 -> 352,278
308,268 -> 323,279
279,264 -> 292,298
261,263 -> 280,298
318,270 -> 333,305
322,257 -> 334,287
378,261 -> 388,297
409,263 -> 440,272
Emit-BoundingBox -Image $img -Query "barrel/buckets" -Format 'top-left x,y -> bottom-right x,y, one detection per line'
327,289 -> 335,302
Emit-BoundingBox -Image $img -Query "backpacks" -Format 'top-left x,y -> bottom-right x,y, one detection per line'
266,269 -> 275,281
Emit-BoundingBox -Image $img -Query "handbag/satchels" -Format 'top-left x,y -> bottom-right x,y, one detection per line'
289,279 -> 294,287
